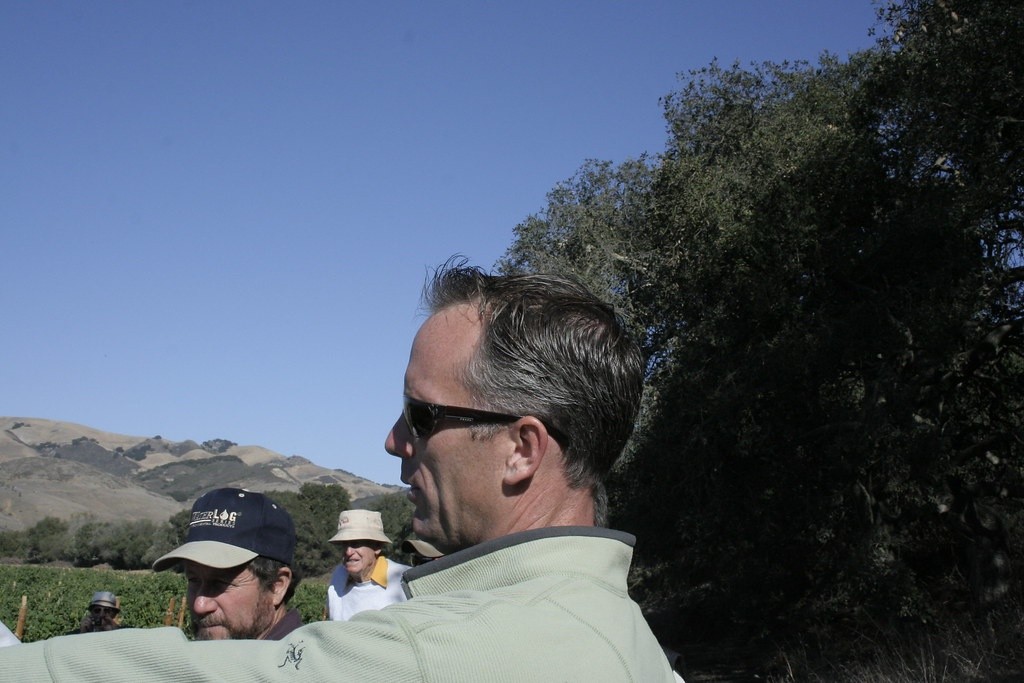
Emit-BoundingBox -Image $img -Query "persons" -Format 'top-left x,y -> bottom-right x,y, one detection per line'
0,256 -> 683,682
66,489 -> 445,642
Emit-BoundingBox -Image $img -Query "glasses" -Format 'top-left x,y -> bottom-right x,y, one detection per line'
411,553 -> 433,567
402,395 -> 568,446
91,608 -> 112,615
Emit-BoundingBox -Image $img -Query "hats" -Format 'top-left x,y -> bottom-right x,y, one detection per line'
88,592 -> 121,615
152,488 -> 296,572
401,539 -> 445,558
328,510 -> 393,543
